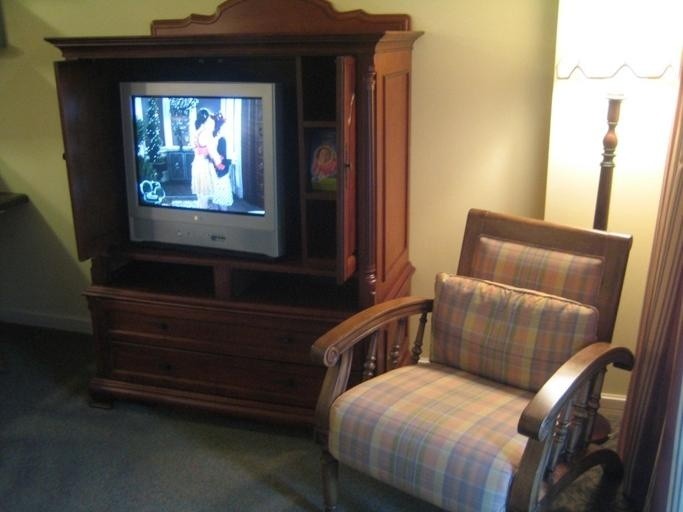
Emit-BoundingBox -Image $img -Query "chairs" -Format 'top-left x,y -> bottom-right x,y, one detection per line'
308,208 -> 640,510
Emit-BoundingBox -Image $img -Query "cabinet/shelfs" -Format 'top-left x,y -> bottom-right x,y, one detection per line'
43,1 -> 425,438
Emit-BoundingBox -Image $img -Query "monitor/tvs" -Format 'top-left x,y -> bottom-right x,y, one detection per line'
117,79 -> 287,259
